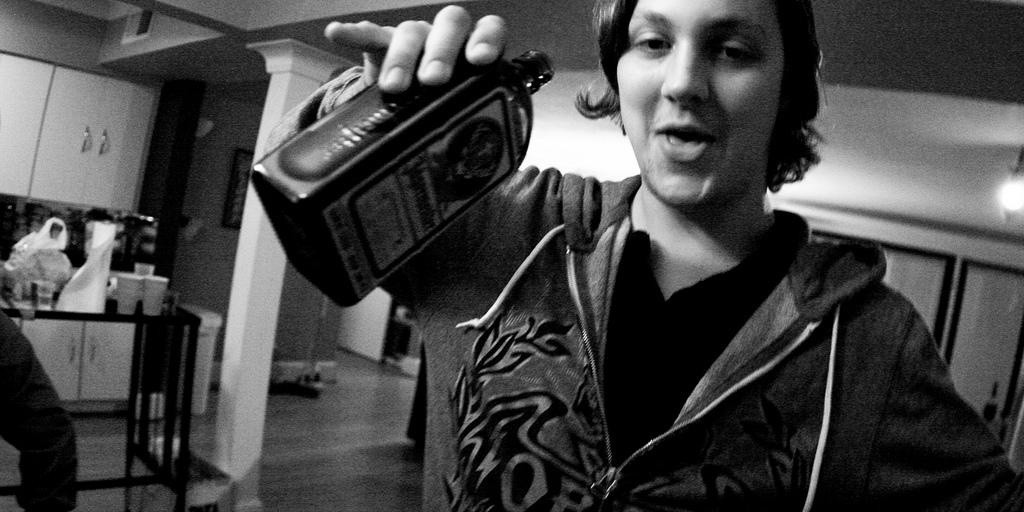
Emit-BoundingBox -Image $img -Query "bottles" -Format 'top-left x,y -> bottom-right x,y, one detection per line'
250,47 -> 554,308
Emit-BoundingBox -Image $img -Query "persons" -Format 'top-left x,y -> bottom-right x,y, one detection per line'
262,0 -> 1023,511
0,305 -> 79,512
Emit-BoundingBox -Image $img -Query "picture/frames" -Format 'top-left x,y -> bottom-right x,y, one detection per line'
220,147 -> 254,229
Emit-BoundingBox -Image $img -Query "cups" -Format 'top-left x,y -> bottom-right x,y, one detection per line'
37,280 -> 55,312
116,262 -> 169,315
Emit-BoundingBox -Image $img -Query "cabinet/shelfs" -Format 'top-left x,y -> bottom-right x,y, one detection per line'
0,49 -> 161,214
19,317 -> 145,420
